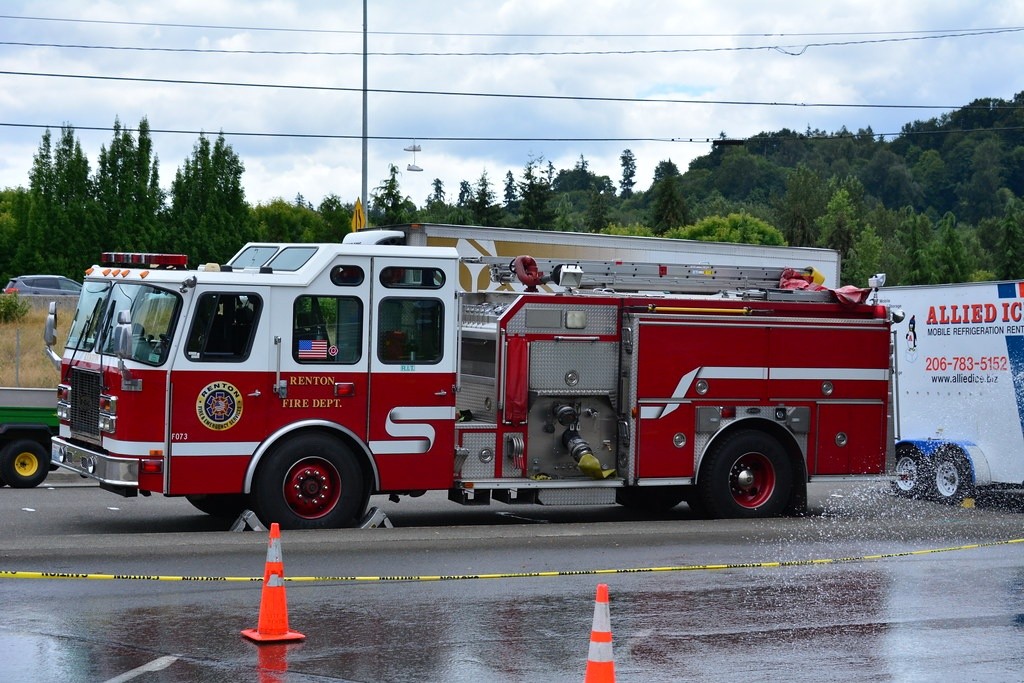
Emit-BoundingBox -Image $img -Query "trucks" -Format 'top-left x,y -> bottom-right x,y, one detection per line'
856,279 -> 1024,504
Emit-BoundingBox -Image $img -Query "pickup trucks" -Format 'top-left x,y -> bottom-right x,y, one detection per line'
0,386 -> 59,488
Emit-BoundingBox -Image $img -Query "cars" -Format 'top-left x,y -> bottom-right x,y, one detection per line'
144,291 -> 176,299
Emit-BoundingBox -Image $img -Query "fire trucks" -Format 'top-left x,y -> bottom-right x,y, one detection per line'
42,220 -> 905,529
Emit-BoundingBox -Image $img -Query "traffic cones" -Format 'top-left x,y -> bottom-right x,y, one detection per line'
584,584 -> 615,683
241,523 -> 305,643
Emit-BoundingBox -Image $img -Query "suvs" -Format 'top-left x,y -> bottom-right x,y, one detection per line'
3,275 -> 83,295
